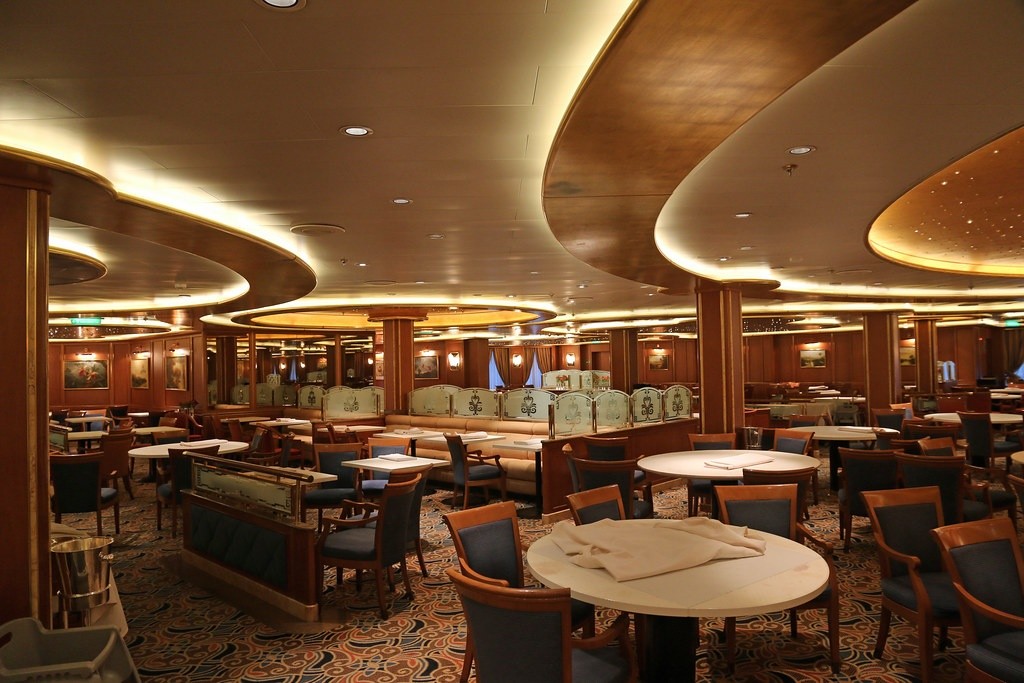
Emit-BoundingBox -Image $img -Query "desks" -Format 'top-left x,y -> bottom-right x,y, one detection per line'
65,415 -> 113,432
218,415 -> 272,443
289,423 -> 386,469
341,453 -> 450,551
67,430 -> 109,454
813,396 -> 866,407
526,519 -> 830,683
127,411 -> 180,429
249,416 -> 311,469
969,392 -> 1021,414
128,439 -> 250,539
130,425 -> 190,484
373,428 -> 506,494
786,426 -> 901,490
636,449 -> 822,520
923,412 -> 1024,461
230,466 -> 338,522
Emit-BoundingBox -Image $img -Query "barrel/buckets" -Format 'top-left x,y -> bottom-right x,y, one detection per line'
49,535 -> 116,629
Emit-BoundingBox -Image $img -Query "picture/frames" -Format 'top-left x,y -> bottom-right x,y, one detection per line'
649,350 -> 827,370
61,355 -> 439,388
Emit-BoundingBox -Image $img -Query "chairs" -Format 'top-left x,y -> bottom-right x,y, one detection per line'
45,371 -> 1024,683
